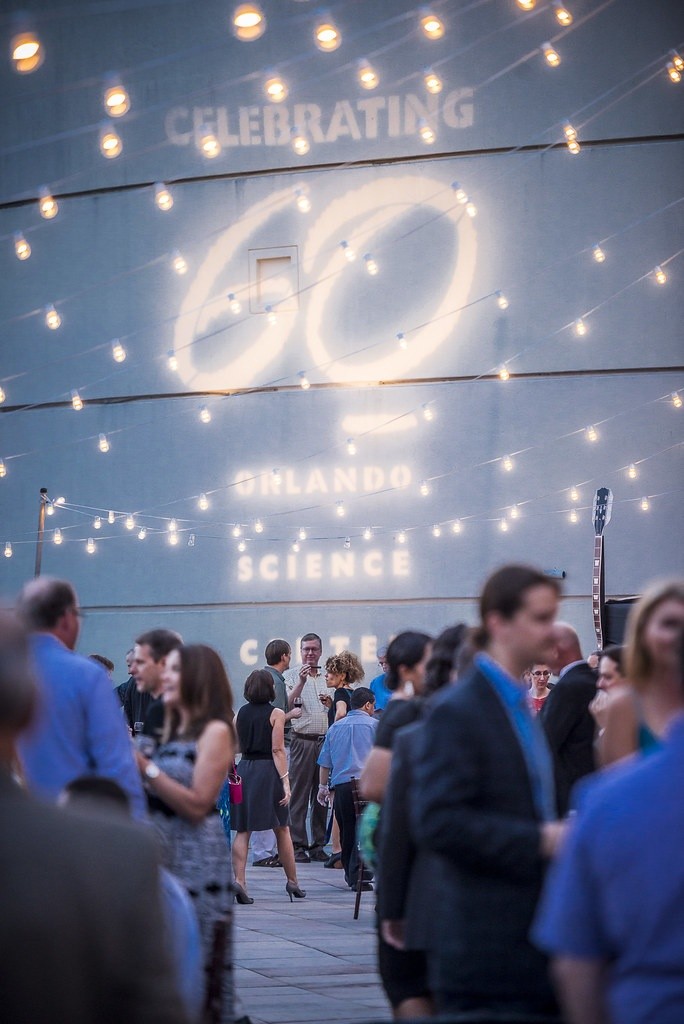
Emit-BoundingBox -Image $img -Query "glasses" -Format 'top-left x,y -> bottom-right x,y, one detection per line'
301,648 -> 321,653
531,671 -> 551,677
378,662 -> 388,666
72,610 -> 87,624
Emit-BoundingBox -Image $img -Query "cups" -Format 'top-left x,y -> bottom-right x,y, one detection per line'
293,697 -> 303,708
136,735 -> 156,761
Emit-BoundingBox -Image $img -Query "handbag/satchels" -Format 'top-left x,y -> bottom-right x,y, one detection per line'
228,774 -> 243,805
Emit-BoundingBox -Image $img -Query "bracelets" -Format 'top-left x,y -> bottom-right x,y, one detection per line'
280,772 -> 289,779
147,761 -> 160,779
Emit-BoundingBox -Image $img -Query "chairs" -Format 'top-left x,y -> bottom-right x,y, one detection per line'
351,776 -> 379,920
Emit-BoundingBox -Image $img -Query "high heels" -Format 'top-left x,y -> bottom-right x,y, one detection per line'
286,881 -> 306,902
232,882 -> 254,904
324,851 -> 343,869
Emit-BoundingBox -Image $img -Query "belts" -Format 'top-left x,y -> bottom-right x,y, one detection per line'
292,732 -> 326,741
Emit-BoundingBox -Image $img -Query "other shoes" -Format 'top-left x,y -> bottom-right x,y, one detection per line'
351,881 -> 373,892
309,850 -> 330,862
361,872 -> 373,880
295,852 -> 311,863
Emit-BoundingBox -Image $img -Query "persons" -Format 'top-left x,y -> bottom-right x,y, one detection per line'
369,646 -> 392,712
318,650 -> 365,870
123,649 -> 149,736
282,632 -> 337,863
250,640 -> 302,867
58,777 -> 209,1024
136,643 -> 237,1024
12,576 -> 146,824
358,565 -> 602,1024
86,654 -> 114,686
130,628 -> 185,758
0,601 -> 194,1024
232,669 -> 307,904
317,687 -> 379,891
530,578 -> 684,1024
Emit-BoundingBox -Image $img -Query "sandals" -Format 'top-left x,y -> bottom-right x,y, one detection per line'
253,854 -> 283,867
